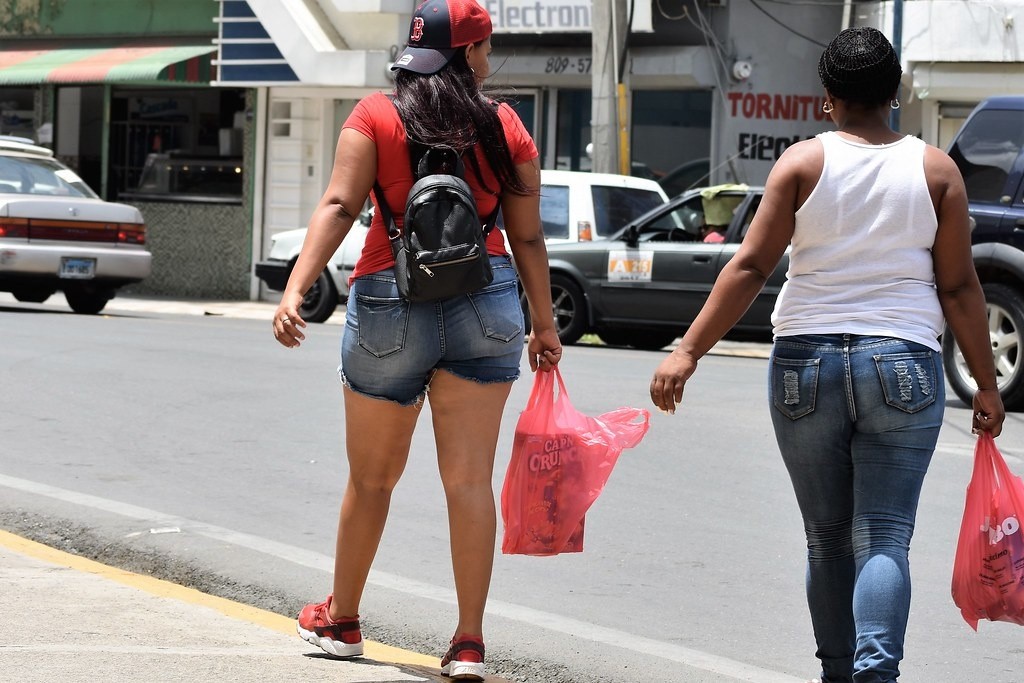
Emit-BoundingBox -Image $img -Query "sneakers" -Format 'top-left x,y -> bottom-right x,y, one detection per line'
441,634 -> 486,680
297,594 -> 364,656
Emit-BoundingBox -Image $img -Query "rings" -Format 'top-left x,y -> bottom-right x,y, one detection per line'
282,317 -> 290,323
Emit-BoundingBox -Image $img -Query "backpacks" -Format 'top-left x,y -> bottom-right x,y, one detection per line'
371,95 -> 504,302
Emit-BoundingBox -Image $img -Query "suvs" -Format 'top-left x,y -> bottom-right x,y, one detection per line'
946,94 -> 1024,412
139,146 -> 242,195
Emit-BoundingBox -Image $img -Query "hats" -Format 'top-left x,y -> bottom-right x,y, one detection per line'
391,0 -> 492,75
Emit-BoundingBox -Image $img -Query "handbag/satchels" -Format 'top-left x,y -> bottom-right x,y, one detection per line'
501,365 -> 651,555
950,432 -> 1024,631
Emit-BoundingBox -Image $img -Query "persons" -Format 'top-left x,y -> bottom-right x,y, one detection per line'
272,0 -> 562,681
650,27 -> 1005,683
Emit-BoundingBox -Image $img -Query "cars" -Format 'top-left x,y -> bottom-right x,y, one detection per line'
252,171 -> 682,322
511,185 -> 792,347
0,134 -> 152,313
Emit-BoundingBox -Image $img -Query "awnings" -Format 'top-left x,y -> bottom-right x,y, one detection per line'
0,43 -> 218,84
